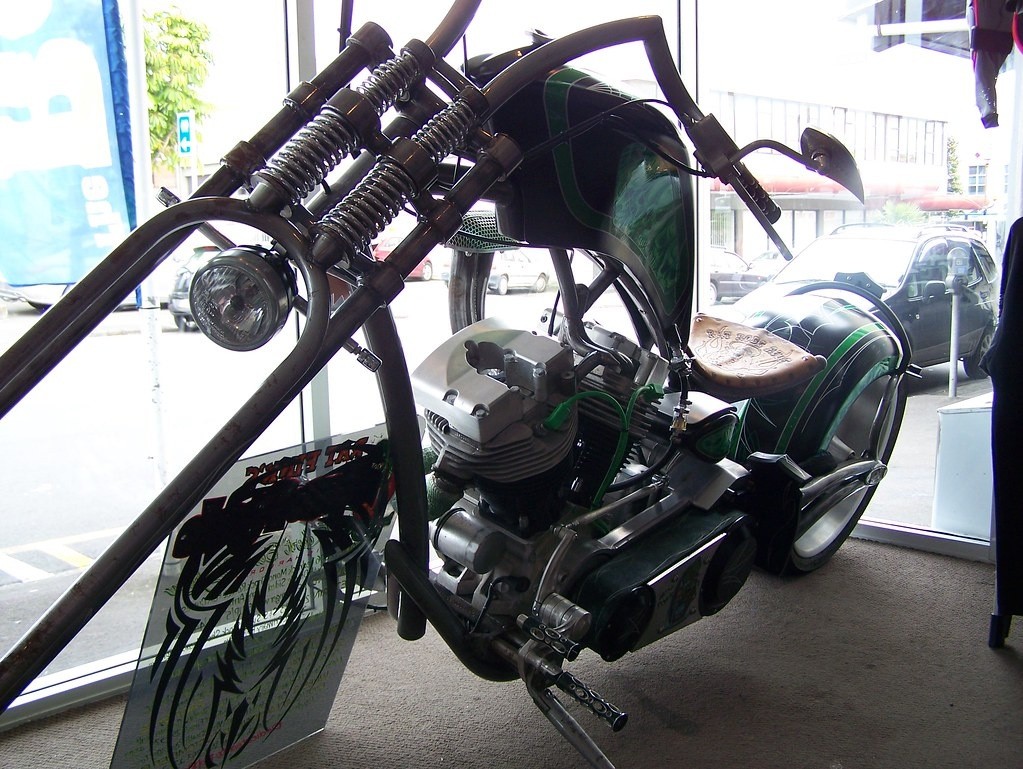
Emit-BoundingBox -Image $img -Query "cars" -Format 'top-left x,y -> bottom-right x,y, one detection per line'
168,244 -> 236,333
442,249 -> 550,296
707,247 -> 771,305
731,219 -> 998,383
368,236 -> 433,282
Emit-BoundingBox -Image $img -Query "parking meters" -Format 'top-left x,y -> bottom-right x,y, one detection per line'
941,247 -> 969,397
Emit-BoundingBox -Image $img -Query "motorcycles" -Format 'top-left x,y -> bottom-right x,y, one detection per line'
0,0 -> 918,769
